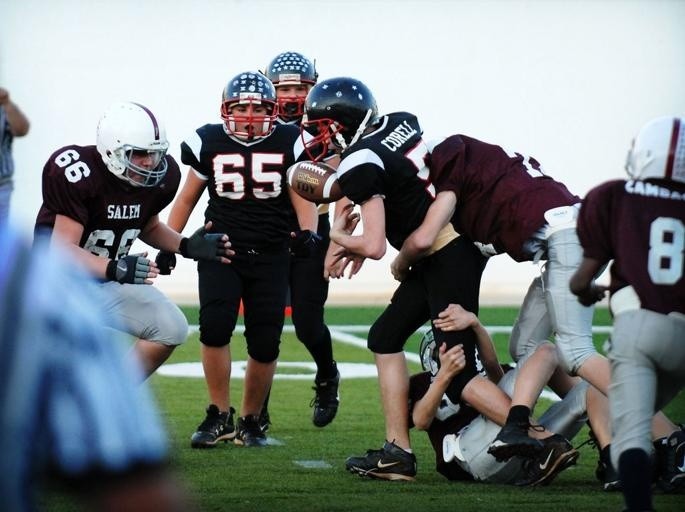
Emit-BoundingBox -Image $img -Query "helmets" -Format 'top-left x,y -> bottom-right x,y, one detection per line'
262,52 -> 319,118
95,101 -> 170,189
624,116 -> 685,184
297,76 -> 379,162
419,323 -> 488,378
219,71 -> 280,141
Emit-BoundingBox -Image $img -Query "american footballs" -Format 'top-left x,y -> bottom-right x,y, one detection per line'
286,160 -> 344,204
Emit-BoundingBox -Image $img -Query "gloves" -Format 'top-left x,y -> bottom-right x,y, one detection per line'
179,226 -> 226,263
106,252 -> 150,285
154,250 -> 176,275
286,228 -> 322,260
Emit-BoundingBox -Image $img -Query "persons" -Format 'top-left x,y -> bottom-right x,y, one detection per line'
569,117 -> 685,512
32,102 -> 236,381
301,78 -> 580,490
390,135 -> 685,495
258,53 -> 352,432
0,219 -> 191,512
155,72 -> 322,449
0,87 -> 30,223
406,304 -> 625,492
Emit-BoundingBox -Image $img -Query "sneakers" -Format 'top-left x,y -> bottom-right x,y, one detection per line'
649,422 -> 685,494
310,358 -> 341,428
345,440 -> 418,482
595,457 -> 623,492
233,413 -> 269,447
512,437 -> 580,487
190,403 -> 237,448
487,420 -> 545,463
